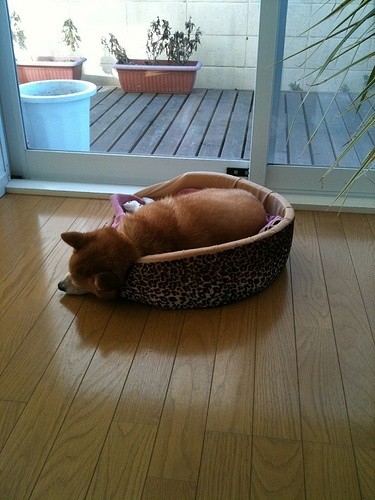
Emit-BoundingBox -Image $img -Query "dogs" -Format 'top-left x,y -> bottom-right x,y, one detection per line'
56,188 -> 267,301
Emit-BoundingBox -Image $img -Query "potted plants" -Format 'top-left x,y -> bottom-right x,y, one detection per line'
10,10 -> 88,84
102,14 -> 204,94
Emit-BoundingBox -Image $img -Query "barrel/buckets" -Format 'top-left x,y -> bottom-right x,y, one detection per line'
17,78 -> 98,153
